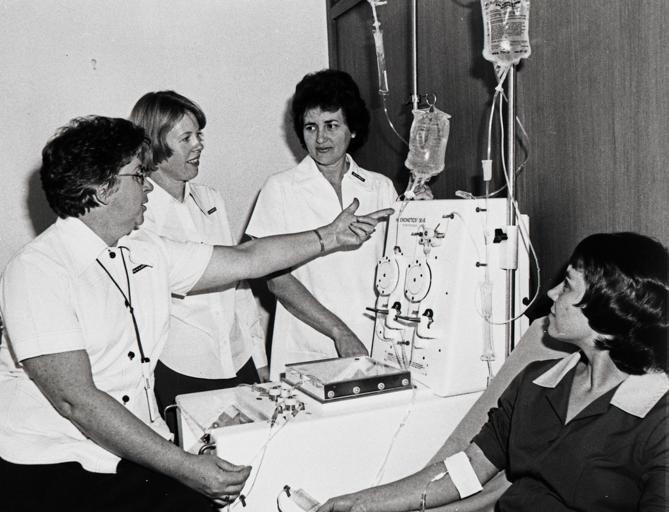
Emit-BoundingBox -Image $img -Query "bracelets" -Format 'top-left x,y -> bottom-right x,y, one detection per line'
313,229 -> 325,256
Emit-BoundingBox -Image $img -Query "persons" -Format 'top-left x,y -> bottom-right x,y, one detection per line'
127,89 -> 269,448
0,113 -> 395,512
316,232 -> 669,512
245,68 -> 403,383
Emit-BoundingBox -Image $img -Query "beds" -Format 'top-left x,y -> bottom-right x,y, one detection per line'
420,314 -> 576,471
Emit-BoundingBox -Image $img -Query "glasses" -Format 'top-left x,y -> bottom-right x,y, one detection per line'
115,169 -> 145,184
